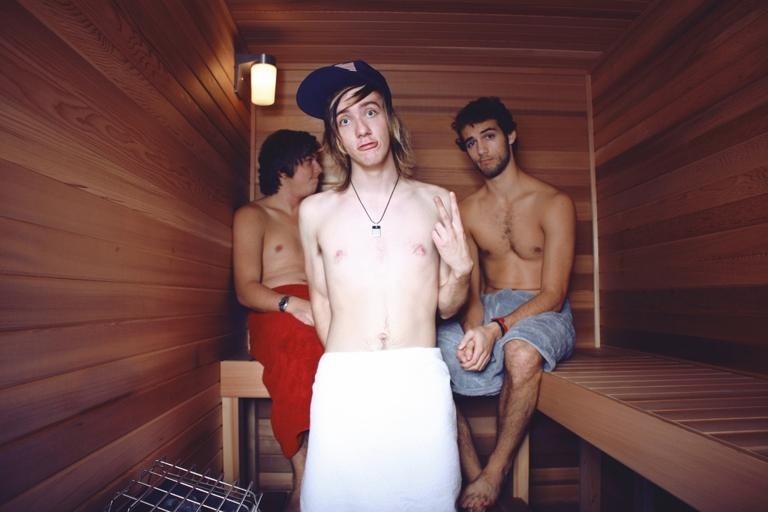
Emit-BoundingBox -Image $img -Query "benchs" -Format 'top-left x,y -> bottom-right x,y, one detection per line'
218,361 -> 601,512
511,342 -> 767,512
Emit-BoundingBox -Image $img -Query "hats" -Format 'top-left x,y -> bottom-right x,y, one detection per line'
296,59 -> 391,122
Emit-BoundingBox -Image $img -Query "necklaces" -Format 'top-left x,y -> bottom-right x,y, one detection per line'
350,173 -> 399,237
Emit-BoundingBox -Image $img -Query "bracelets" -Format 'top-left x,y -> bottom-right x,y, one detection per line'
492,315 -> 508,336
277,294 -> 290,312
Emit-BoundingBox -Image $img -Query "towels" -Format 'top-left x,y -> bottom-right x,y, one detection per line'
300,347 -> 464,512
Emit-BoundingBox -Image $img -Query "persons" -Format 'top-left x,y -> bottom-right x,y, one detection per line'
231,130 -> 325,511
294,58 -> 474,512
434,97 -> 575,512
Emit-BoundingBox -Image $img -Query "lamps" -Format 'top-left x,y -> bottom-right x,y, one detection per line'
233,51 -> 277,107
